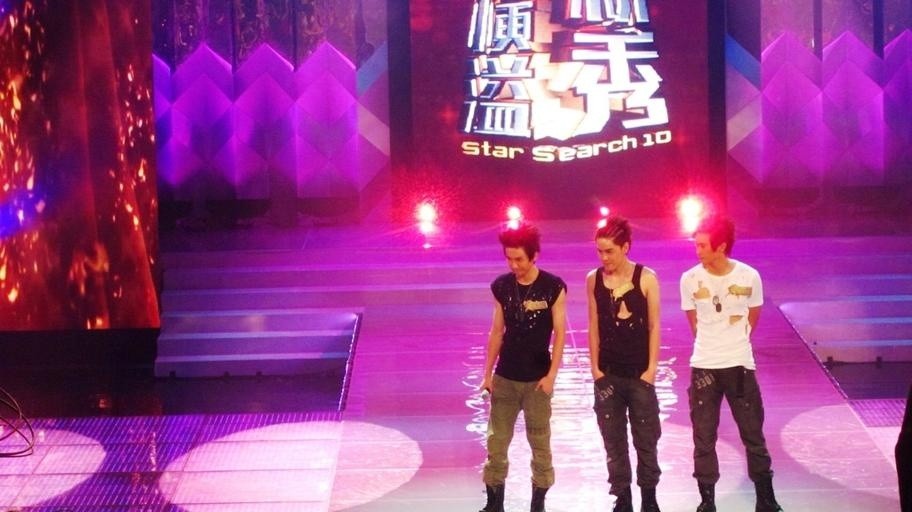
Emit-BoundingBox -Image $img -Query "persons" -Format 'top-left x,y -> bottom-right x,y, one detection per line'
585,214 -> 663,512
477,223 -> 567,512
680,214 -> 784,512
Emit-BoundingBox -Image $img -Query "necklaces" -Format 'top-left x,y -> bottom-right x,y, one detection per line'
515,282 -> 533,312
